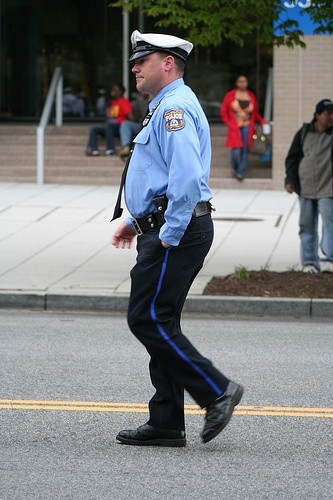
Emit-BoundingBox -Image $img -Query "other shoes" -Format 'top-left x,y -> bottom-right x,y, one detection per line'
106,149 -> 115,156
321,261 -> 333,273
119,146 -> 130,155
87,148 -> 99,157
304,264 -> 317,274
232,168 -> 244,181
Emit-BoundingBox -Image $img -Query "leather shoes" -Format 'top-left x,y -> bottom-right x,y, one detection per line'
202,381 -> 243,444
115,422 -> 186,447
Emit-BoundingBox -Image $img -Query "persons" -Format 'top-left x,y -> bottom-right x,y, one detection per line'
64,83 -> 149,157
113,31 -> 243,447
220,74 -> 269,179
285,99 -> 333,274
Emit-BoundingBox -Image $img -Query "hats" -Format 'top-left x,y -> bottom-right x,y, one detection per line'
317,100 -> 333,113
128,30 -> 193,63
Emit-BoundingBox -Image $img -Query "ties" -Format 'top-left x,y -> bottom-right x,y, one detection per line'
110,108 -> 149,220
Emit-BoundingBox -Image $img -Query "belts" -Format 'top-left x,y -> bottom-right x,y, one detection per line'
132,201 -> 211,235
